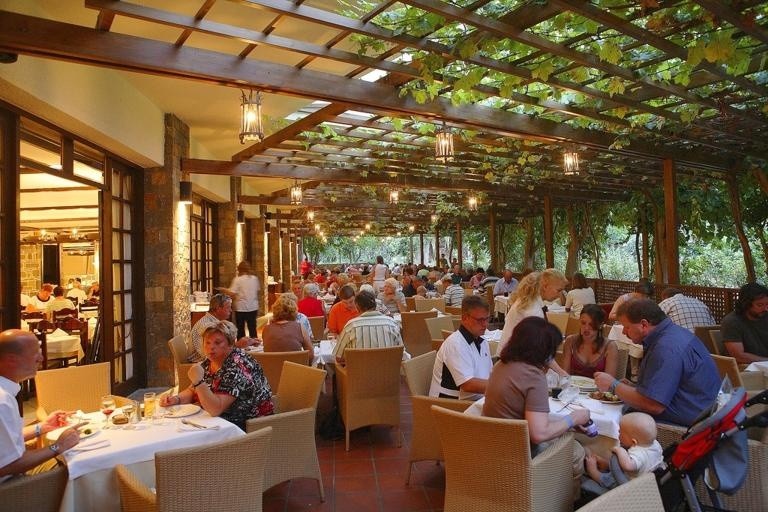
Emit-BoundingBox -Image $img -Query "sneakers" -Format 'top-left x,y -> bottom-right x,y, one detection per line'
576,419 -> 598,438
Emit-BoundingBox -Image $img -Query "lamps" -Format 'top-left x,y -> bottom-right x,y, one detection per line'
291,179 -> 302,206
238,89 -> 264,143
435,122 -> 455,165
563,143 -> 580,176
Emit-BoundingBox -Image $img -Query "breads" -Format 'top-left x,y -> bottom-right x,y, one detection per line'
111,413 -> 129,425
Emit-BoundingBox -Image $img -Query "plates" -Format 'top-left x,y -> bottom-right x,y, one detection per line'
586,393 -> 623,404
158,404 -> 202,418
572,382 -> 600,392
45,424 -> 99,440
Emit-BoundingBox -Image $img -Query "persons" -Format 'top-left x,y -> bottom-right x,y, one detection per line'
427,296 -> 495,401
478,315 -> 596,460
185,293 -> 261,364
585,411 -> 667,492
157,322 -> 277,434
592,297 -> 720,429
261,252 -> 653,381
20,277 -> 106,323
715,282 -> 767,368
214,259 -> 262,346
0,330 -> 90,483
658,287 -> 717,335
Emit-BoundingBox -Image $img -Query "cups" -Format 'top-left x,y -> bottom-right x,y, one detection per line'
546,372 -> 579,404
143,393 -> 157,423
327,332 -> 335,341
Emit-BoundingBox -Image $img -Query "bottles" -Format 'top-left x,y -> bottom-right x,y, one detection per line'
130,400 -> 142,421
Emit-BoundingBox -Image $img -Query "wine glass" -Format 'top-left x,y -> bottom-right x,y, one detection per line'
100,395 -> 115,429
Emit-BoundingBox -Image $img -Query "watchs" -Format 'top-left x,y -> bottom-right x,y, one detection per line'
50,444 -> 59,453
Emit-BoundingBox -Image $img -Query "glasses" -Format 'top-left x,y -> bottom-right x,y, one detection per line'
466,314 -> 490,323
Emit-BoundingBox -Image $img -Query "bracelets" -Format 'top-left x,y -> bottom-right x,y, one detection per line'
173,395 -> 180,405
562,413 -> 574,432
608,378 -> 619,397
191,379 -> 205,388
35,422 -> 42,436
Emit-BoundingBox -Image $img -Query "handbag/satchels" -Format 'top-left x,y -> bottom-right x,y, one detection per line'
319,406 -> 343,441
